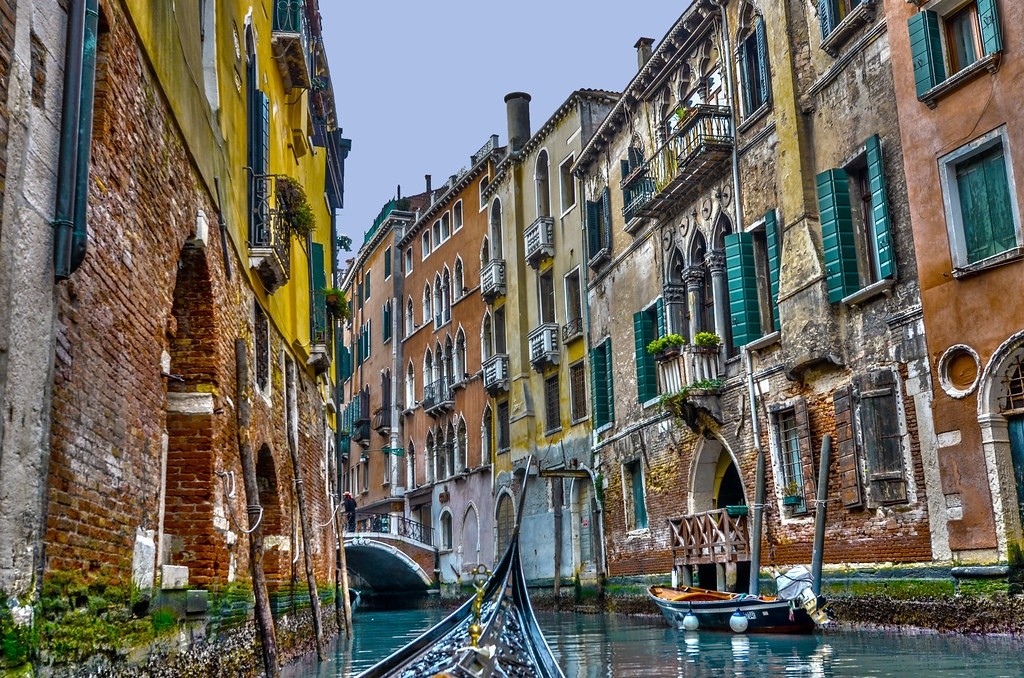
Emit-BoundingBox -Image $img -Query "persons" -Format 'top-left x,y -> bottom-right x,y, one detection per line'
343,491 -> 357,532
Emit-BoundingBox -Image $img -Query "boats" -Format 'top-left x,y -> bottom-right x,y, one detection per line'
647,582 -> 826,631
352,452 -> 567,678
